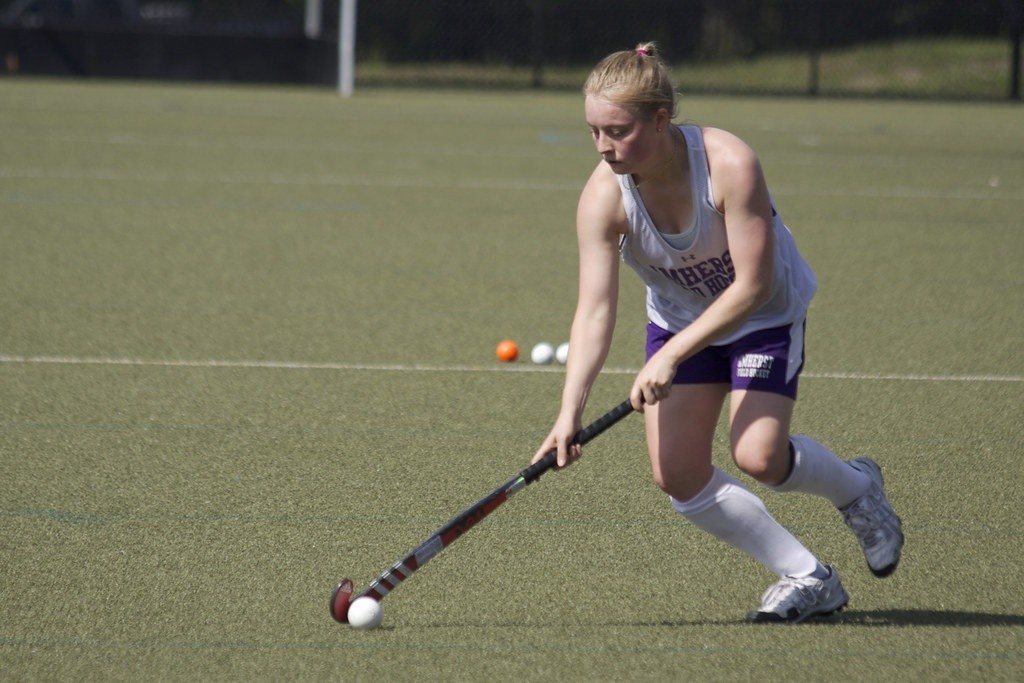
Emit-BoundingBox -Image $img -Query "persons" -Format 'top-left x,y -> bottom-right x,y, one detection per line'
530,42 -> 905,624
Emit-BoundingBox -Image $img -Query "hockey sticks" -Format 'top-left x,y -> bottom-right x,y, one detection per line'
331,392 -> 647,624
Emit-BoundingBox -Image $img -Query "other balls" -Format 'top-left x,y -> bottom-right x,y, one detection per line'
495,339 -> 520,363
556,344 -> 569,365
347,595 -> 383,630
532,342 -> 555,366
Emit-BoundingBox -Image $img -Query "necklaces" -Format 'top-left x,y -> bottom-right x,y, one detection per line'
622,126 -> 678,190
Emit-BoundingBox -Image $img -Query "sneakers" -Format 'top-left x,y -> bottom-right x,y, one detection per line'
838,458 -> 904,576
746,564 -> 849,623
